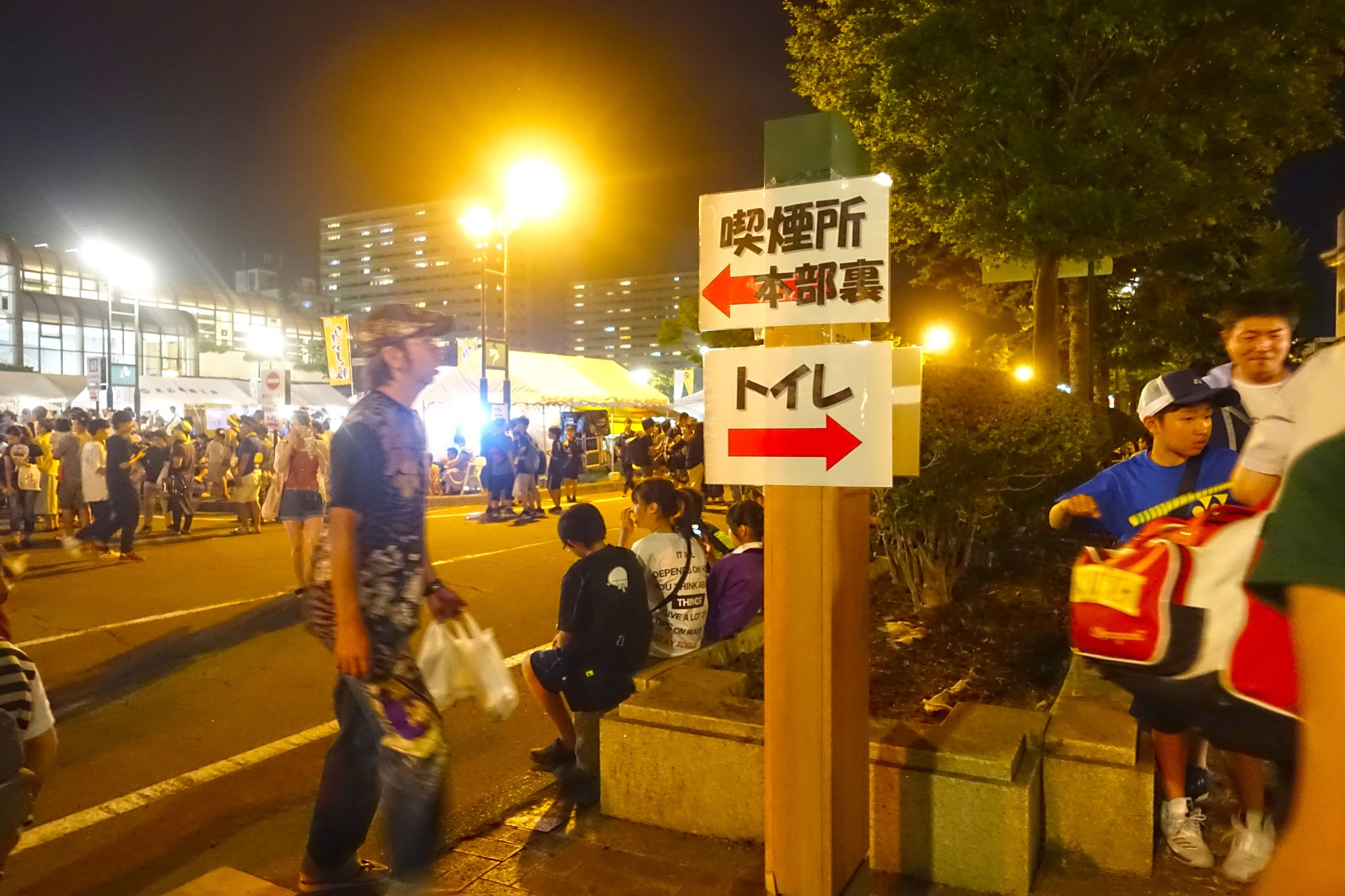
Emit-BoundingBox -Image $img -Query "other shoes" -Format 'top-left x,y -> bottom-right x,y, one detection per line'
548,506 -> 562,513
493,509 -> 518,518
230,526 -> 261,534
138,525 -> 151,534
97,550 -> 120,559
710,501 -> 726,505
517,508 -> 546,520
529,738 -> 576,765
118,549 -> 146,563
167,529 -> 190,535
566,496 -> 576,502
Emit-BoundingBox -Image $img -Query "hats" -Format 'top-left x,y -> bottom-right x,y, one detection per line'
1136,371 -> 1241,423
226,413 -> 241,427
169,421 -> 193,443
358,302 -> 457,357
642,416 -> 658,431
71,411 -> 91,426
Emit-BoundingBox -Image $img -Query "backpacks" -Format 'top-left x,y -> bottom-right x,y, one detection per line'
556,444 -> 575,476
625,436 -> 650,467
523,434 -> 546,475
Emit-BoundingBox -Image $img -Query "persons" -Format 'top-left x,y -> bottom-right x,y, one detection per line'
423,434 -> 477,497
1179,296 -> 1308,802
0,541 -> 59,831
89,410 -> 146,563
613,411 -> 765,508
545,425 -> 568,513
478,415 -> 546,520
0,405 -> 333,544
561,425 -> 587,503
60,418 -> 122,559
1241,431 -> 1345,896
674,486 -> 765,641
1047,371 -> 1276,882
617,477 -> 709,663
273,409 -> 330,599
297,303 -> 471,896
1227,337 -> 1345,838
521,502 -> 654,764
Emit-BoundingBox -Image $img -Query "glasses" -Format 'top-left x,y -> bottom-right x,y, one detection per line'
563,542 -> 571,552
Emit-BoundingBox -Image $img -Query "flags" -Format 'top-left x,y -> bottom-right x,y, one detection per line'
682,369 -> 694,395
673,370 -> 685,401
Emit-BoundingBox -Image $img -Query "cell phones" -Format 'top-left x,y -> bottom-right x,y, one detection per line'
691,524 -> 702,535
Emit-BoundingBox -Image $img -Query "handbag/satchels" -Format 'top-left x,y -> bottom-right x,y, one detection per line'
261,471 -> 283,520
1069,505 -> 1306,726
416,610 -> 519,717
162,466 -> 186,499
17,464 -> 42,491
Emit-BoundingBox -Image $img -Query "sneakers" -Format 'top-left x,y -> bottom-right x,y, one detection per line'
1160,795 -> 1278,882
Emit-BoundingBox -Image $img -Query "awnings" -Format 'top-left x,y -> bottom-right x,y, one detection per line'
126,375 -> 353,408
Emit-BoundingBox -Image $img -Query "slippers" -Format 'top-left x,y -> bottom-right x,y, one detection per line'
300,858 -> 391,892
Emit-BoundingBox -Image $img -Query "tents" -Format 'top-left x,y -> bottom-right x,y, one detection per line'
0,370 -> 89,410
667,389 -> 704,423
409,337 -> 670,475
